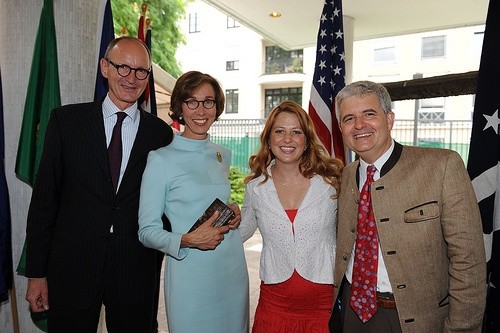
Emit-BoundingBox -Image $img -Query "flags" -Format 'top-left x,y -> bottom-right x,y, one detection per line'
468,0 -> 500,333
94,0 -> 116,101
15,0 -> 61,193
136,16 -> 157,115
306,0 -> 348,171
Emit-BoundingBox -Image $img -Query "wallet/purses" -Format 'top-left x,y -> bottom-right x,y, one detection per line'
188,198 -> 234,234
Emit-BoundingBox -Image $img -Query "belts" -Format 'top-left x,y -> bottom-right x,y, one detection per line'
376,293 -> 396,309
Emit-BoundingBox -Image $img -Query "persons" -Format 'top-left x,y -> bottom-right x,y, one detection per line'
15,36 -> 174,333
327,81 -> 487,333
139,71 -> 250,333
236,101 -> 344,333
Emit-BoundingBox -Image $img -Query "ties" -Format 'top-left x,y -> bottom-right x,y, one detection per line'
349,165 -> 380,324
107,111 -> 128,193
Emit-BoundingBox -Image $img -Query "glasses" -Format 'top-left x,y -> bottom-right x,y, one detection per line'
106,57 -> 152,80
183,98 -> 216,109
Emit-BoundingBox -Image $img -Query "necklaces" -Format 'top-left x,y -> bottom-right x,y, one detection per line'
274,167 -> 301,185
